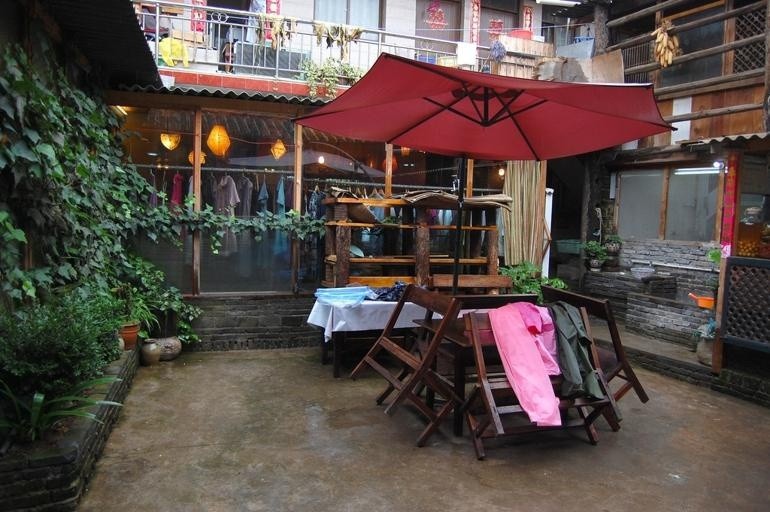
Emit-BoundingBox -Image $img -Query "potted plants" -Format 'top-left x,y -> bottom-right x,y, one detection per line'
118,297 -> 160,350
582,236 -> 623,268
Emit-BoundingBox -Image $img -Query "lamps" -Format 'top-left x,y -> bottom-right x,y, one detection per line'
160,124 -> 286,167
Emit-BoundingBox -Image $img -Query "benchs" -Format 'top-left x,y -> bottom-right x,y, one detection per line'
454,293 -> 538,307
458,306 -> 623,457
539,284 -> 648,443
352,283 -> 461,446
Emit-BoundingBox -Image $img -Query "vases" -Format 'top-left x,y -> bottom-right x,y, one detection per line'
141,339 -> 160,366
150,335 -> 182,361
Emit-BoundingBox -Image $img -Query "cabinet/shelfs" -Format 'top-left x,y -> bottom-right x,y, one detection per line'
321,198 -> 513,295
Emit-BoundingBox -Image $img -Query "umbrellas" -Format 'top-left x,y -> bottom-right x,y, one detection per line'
288,51 -> 678,296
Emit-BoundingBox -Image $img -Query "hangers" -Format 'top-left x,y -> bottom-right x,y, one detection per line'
148,162 -> 503,204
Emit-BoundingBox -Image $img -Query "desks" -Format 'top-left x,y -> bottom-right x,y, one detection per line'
412,316 -> 470,437
306,297 -> 444,378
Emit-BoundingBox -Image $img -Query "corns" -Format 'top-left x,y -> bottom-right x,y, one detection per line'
654,27 -> 679,68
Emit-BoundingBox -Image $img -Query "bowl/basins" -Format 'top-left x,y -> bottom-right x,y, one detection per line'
631,267 -> 655,279
